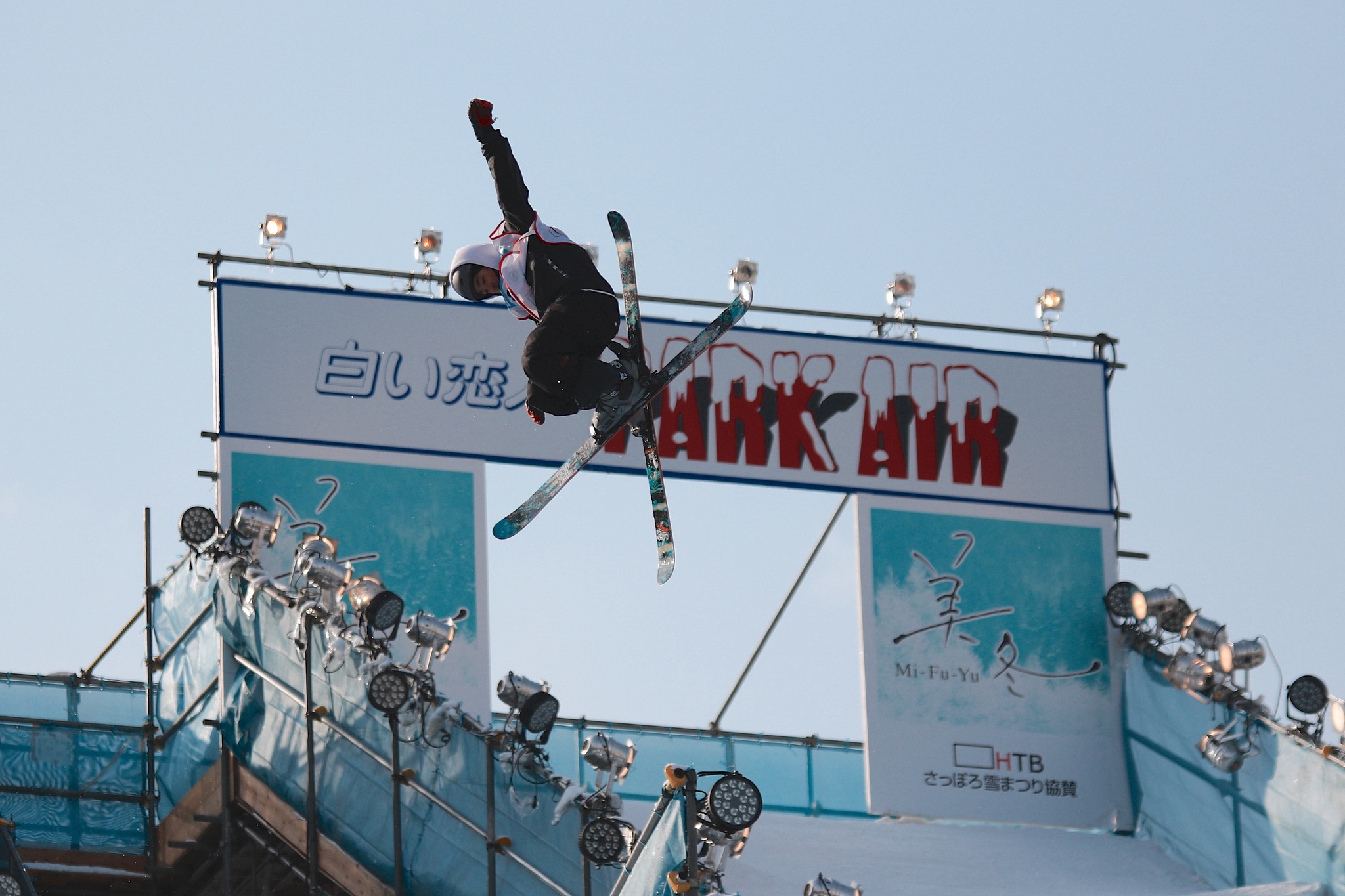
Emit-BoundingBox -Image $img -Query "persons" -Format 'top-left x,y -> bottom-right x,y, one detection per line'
447,99 -> 645,441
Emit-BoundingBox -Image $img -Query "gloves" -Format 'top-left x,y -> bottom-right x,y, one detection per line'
524,399 -> 545,425
468,99 -> 496,137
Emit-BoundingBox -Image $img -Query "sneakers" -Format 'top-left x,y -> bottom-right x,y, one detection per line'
588,372 -> 650,440
613,359 -> 647,438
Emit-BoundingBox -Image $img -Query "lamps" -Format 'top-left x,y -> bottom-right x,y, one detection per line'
888,274 -> 914,318
493,672 -> 559,752
303,557 -> 352,619
804,873 -> 862,896
259,214 -> 289,261
414,227 -> 443,275
287,535 -> 335,594
177,505 -> 224,556
1035,287 -> 1064,334
405,612 -> 457,687
229,504 -> 280,565
582,242 -> 600,266
578,816 -> 639,870
1102,577 -> 1345,772
340,581 -> 403,654
732,260 -> 757,294
582,731 -> 637,807
361,662 -> 420,720
692,768 -> 764,841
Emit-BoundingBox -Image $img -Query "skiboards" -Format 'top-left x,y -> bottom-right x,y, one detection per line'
490,210 -> 754,584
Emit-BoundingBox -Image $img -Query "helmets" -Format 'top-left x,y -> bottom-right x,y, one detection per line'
452,263 -> 481,301
